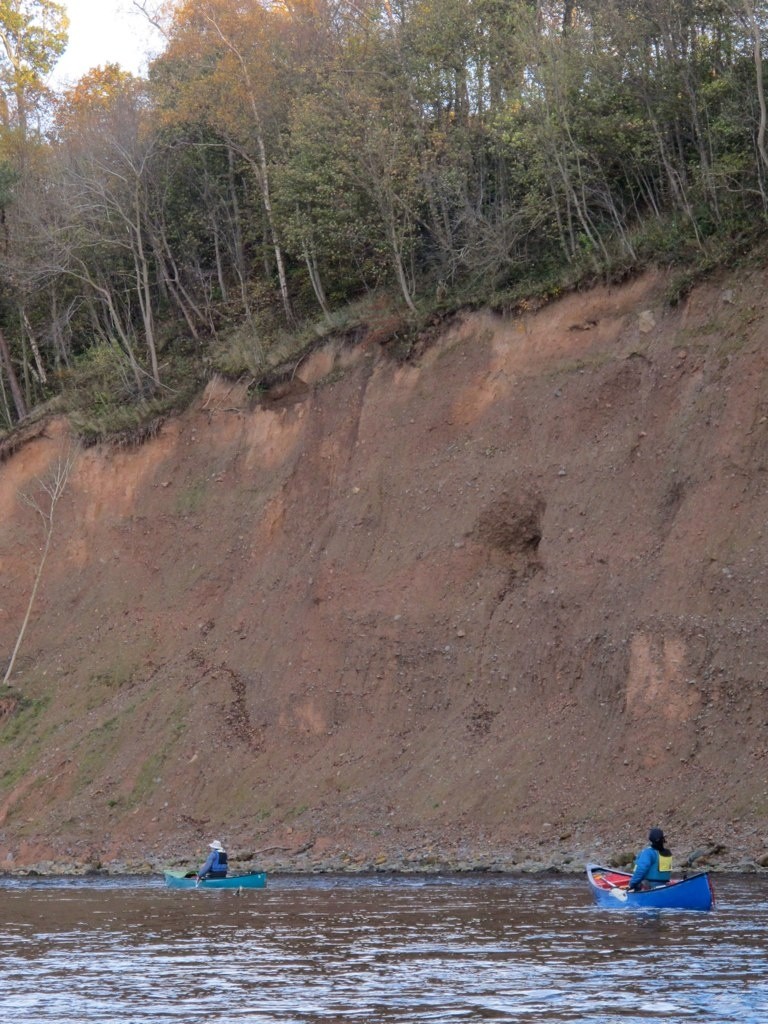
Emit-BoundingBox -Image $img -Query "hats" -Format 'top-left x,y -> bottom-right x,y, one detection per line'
649,829 -> 664,840
209,840 -> 222,849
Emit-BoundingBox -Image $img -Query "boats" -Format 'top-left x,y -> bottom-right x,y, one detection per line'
584,861 -> 713,911
163,870 -> 267,889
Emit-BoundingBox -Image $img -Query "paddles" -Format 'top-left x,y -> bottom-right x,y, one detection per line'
196,877 -> 202,887
611,886 -> 630,902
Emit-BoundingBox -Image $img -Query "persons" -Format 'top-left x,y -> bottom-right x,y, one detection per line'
197,839 -> 228,879
625,828 -> 673,892
